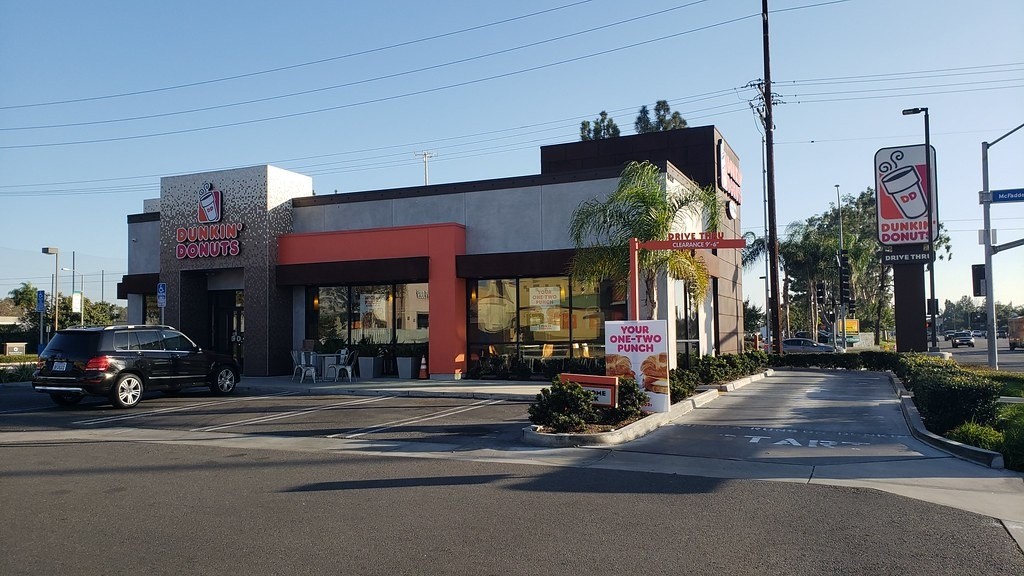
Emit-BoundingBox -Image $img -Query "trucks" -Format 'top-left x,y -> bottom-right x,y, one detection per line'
1007,316 -> 1024,350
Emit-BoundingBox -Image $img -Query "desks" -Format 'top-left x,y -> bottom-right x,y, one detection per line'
311,353 -> 344,382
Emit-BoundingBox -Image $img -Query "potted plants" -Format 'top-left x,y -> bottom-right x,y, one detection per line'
395,336 -> 419,379
357,335 -> 384,380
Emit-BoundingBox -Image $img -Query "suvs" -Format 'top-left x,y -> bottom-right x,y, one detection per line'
32,324 -> 242,410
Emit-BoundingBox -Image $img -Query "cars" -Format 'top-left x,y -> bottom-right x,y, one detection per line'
764,338 -> 847,353
962,327 -> 1009,340
944,330 -> 956,341
795,330 -> 832,344
951,332 -> 974,348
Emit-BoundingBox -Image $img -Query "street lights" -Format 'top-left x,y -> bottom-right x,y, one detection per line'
62,268 -> 84,325
902,104 -> 941,352
43,247 -> 59,333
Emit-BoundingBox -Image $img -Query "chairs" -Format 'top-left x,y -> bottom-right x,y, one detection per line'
335,350 -> 359,383
291,348 -> 303,380
299,350 -> 322,384
326,348 -> 350,383
